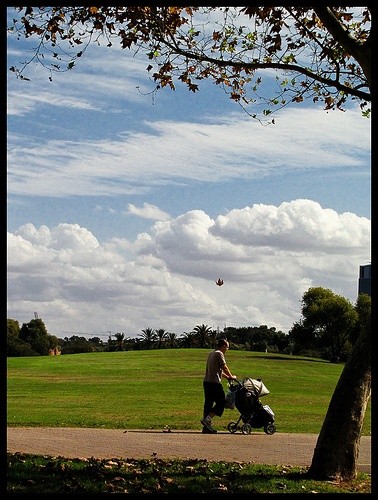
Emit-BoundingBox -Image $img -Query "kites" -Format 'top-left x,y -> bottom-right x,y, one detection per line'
215,278 -> 224,287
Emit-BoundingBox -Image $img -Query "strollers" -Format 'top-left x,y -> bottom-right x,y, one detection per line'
226,377 -> 276,436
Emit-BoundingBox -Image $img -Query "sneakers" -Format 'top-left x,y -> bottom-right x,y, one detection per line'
201,427 -> 218,434
201,418 -> 215,434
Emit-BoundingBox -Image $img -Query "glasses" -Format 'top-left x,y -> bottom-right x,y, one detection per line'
226,346 -> 230,349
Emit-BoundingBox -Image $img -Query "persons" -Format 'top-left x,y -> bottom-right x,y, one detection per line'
201,339 -> 236,434
